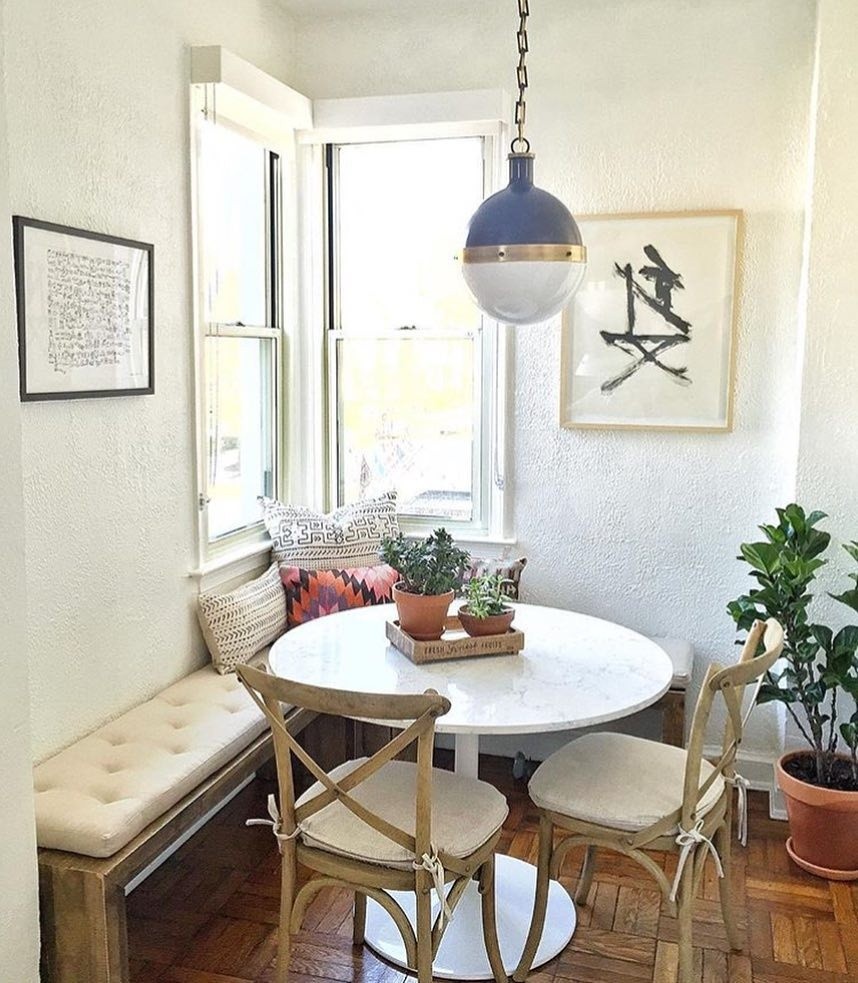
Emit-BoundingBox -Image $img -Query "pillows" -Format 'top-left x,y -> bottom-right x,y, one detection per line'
195,558 -> 290,675
257,488 -> 408,567
398,545 -> 528,603
279,564 -> 399,632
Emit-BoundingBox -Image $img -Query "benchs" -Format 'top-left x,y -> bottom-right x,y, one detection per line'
28,640 -> 695,983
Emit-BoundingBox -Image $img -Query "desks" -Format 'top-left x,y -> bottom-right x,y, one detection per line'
266,598 -> 676,983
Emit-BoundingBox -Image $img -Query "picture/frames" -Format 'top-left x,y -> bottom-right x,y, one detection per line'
557,208 -> 744,437
10,214 -> 155,402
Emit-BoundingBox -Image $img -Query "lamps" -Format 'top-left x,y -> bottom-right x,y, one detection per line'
462,0 -> 586,327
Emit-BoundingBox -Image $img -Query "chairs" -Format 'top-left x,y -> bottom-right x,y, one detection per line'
510,616 -> 786,981
232,660 -> 510,983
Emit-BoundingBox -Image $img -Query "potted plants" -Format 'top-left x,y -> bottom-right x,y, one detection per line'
382,529 -> 467,644
730,501 -> 858,881
456,573 -> 517,640
